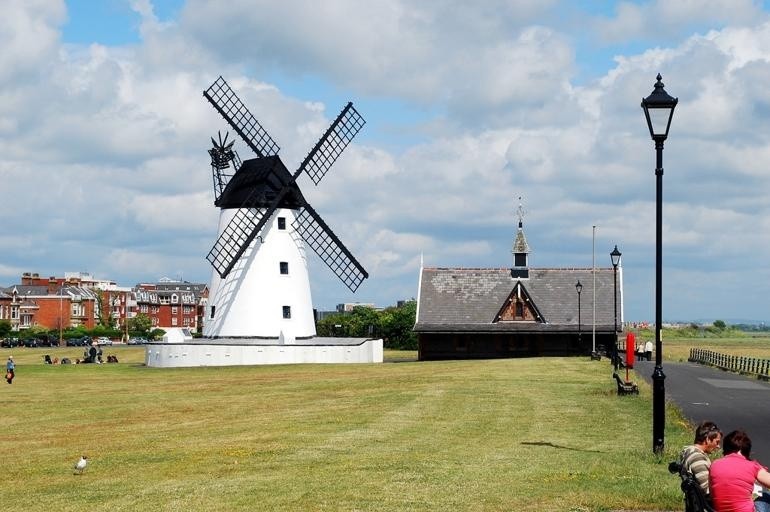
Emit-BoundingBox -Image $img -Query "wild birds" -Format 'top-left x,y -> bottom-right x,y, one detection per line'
72,454 -> 89,475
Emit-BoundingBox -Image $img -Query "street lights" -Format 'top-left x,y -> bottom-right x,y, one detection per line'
641,73 -> 677,453
610,246 -> 622,371
576,281 -> 583,356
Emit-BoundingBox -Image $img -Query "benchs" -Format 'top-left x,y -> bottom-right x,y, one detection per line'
612,372 -> 639,396
668,461 -> 716,512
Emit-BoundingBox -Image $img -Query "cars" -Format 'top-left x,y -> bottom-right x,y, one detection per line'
66,336 -> 112,346
128,337 -> 143,345
0,337 -> 43,347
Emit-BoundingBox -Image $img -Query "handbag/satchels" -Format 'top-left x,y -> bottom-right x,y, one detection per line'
5,373 -> 12,380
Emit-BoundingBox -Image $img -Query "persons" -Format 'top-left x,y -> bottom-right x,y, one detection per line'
6,356 -> 16,384
682,421 -> 723,509
637,343 -> 645,361
646,340 -> 653,361
708,429 -> 770,512
46,345 -> 118,364
